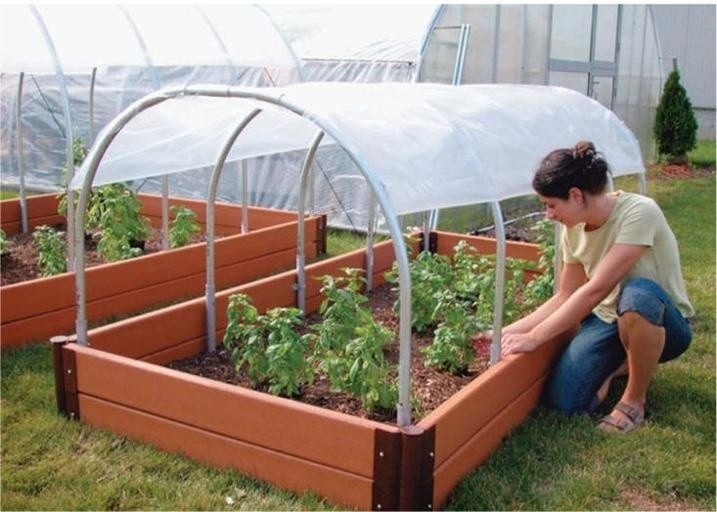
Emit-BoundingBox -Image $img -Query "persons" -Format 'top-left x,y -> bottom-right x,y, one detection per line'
478,141 -> 695,437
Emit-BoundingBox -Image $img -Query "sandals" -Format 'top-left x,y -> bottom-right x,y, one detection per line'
594,400 -> 647,436
